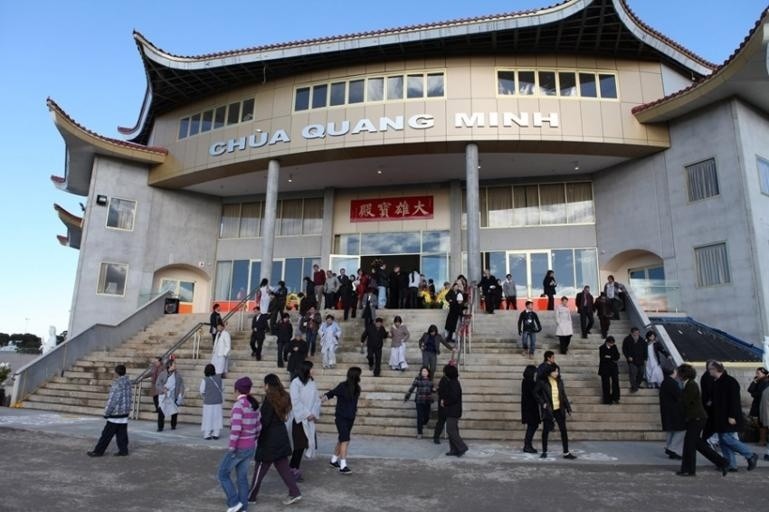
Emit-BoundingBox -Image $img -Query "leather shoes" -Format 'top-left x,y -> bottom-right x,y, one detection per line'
676,469 -> 696,478
330,461 -> 340,470
722,460 -> 730,477
112,451 -> 128,457
540,452 -> 547,458
339,466 -> 353,474
746,452 -> 759,471
86,448 -> 103,457
562,452 -> 577,460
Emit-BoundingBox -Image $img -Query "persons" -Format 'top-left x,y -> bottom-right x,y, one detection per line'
376,262 -> 389,308
658,361 -> 688,460
210,323 -> 231,378
283,332 -> 308,362
701,359 -> 719,443
360,317 -> 390,377
474,269 -> 498,314
266,281 -> 287,335
534,362 -> 578,459
256,279 -> 271,320
593,291 -> 615,338
419,325 -> 456,379
323,270 -> 340,309
249,307 -> 266,360
746,367 -> 769,442
388,316 -> 409,370
357,269 -> 368,309
676,364 -> 731,477
403,367 -> 436,439
343,275 -> 358,321
442,365 -> 469,457
303,277 -> 317,308
320,366 -> 362,475
575,285 -> 594,338
494,279 -> 503,309
389,266 -> 401,308
428,279 -> 435,308
313,264 -> 326,308
209,304 -> 224,344
518,301 -> 542,358
521,365 -> 541,453
217,378 -> 261,512
276,313 -> 293,368
318,313 -> 342,369
598,336 -> 621,405
87,365 -> 131,456
248,374 -> 302,505
285,361 -> 321,482
537,351 -> 560,431
702,360 -> 758,471
303,307 -> 322,358
286,347 -> 305,381
363,265 -> 378,307
148,357 -> 164,412
200,364 -> 223,440
360,288 -> 377,354
437,282 -> 451,308
417,274 -> 432,308
622,327 -> 649,393
540,270 -> 557,310
503,274 -> 517,310
433,366 -> 449,445
555,296 -> 573,355
455,275 -> 473,291
444,280 -> 468,342
644,331 -> 672,389
155,359 -> 185,431
336,268 -> 349,308
409,267 -> 419,308
297,291 -> 313,334
604,276 -> 621,320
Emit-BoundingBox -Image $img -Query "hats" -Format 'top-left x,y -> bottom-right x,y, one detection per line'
234,376 -> 253,395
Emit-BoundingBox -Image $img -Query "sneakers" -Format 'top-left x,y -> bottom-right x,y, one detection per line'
289,468 -> 305,482
225,502 -> 243,512
282,494 -> 303,506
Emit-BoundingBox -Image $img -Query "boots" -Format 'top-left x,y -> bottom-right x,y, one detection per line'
523,438 -> 538,453
